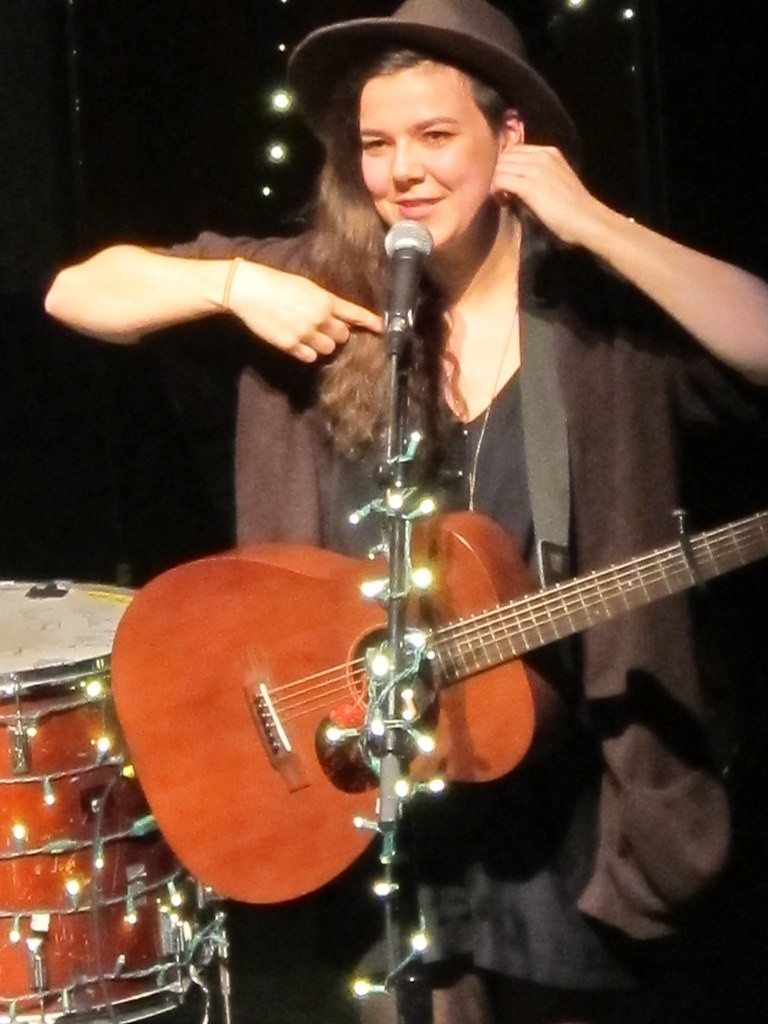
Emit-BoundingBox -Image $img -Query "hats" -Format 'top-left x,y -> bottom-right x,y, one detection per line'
286,0 -> 577,162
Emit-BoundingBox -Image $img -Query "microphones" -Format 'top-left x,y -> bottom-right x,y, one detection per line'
384,220 -> 434,358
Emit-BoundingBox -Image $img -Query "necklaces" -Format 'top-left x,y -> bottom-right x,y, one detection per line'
458,306 -> 516,514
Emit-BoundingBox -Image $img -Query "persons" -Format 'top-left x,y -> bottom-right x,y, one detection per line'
45,0 -> 768,1024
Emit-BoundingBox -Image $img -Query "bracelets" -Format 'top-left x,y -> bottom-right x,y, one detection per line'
222,257 -> 244,310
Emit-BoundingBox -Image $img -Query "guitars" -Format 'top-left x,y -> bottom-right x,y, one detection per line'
111,509 -> 768,904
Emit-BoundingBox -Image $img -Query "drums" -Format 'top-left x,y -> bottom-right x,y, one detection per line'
1,580 -> 229,1021
